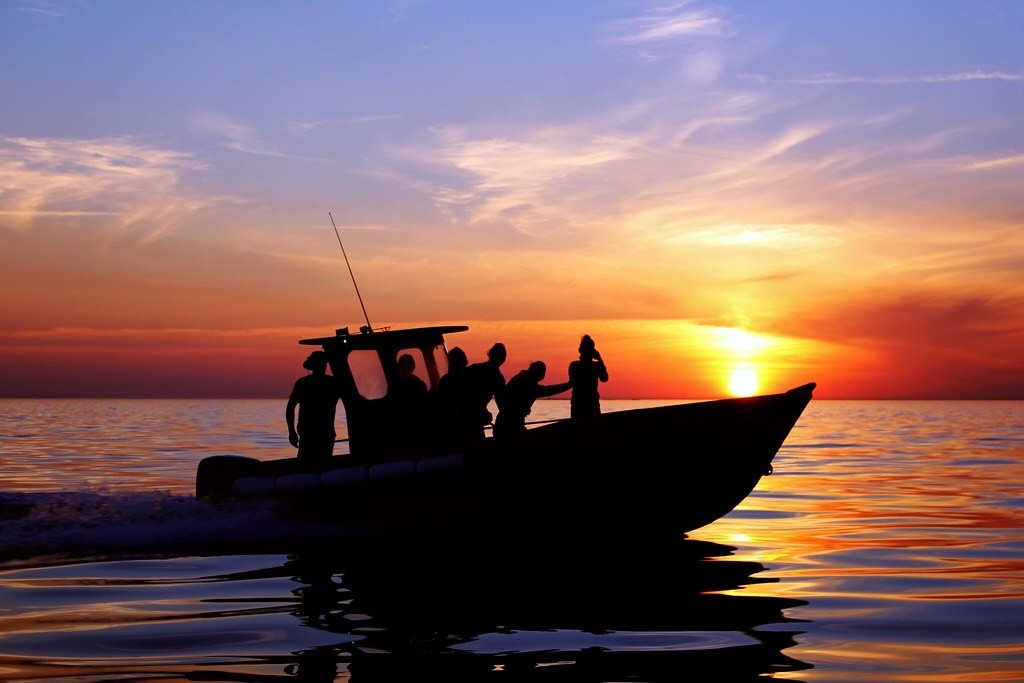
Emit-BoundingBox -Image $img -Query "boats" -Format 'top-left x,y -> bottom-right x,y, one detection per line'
191,322 -> 817,553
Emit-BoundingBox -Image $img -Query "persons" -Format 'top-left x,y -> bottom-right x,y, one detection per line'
285,333 -> 609,458
